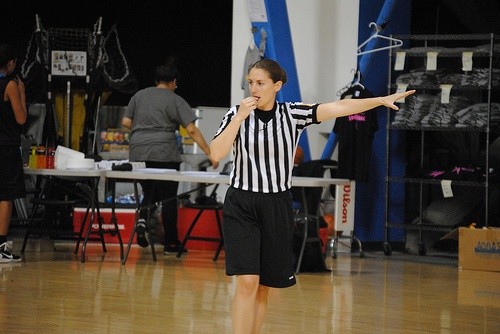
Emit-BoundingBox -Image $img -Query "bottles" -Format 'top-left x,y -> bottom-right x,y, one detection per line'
37,143 -> 47,169
28,143 -> 38,169
46,147 -> 54,169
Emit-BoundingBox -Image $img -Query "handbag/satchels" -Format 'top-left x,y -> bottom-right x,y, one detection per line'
457,226 -> 500,307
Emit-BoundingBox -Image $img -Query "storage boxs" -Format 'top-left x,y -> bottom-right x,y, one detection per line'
457,226 -> 500,273
458,270 -> 500,309
72,207 -> 137,243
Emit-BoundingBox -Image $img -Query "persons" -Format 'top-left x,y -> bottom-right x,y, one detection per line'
290,146 -> 319,215
121,66 -> 211,254
0,46 -> 28,263
209,59 -> 416,334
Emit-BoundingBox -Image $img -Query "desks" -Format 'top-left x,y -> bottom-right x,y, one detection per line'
106,170 -> 350,274
23,168 -> 111,263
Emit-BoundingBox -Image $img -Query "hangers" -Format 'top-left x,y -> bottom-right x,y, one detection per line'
336,68 -> 364,97
250,33 -> 255,49
352,22 -> 403,56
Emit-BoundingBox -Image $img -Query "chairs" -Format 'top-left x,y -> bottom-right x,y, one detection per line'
21,160 -> 339,272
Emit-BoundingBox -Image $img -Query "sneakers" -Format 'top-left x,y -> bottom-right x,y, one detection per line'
164,242 -> 188,254
0,239 -> 22,262
135,218 -> 149,247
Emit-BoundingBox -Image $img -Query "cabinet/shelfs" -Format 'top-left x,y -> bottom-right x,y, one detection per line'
384,35 -> 500,255
